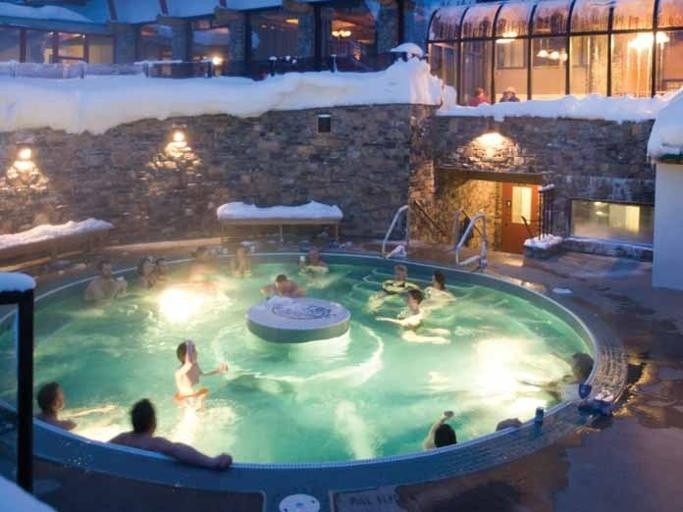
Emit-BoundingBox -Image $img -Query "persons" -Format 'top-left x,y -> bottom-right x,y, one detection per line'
173,338 -> 229,400
514,350 -> 595,410
499,87 -> 520,102
422,411 -> 521,451
106,395 -> 232,472
467,85 -> 492,108
34,381 -> 115,429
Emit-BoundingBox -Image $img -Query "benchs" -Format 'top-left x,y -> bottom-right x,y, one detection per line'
217,216 -> 341,245
0,228 -> 105,269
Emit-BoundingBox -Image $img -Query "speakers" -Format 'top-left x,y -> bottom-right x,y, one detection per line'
317,115 -> 332,135
